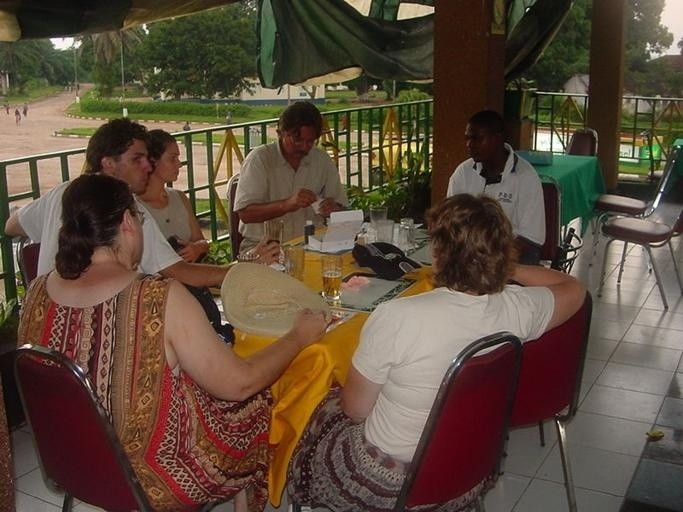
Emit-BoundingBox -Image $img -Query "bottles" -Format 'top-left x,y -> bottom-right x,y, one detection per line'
303,220 -> 314,244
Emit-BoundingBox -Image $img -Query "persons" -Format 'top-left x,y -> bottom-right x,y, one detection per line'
446,109 -> 547,263
17,172 -> 328,511
4,100 -> 10,114
3,117 -> 280,288
14,108 -> 20,123
285,194 -> 588,512
23,102 -> 28,117
232,99 -> 352,256
135,129 -> 235,326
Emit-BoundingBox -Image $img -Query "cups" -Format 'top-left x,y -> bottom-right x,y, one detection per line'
355,208 -> 414,255
321,256 -> 343,300
263,219 -> 283,246
284,246 -> 305,283
236,253 -> 262,265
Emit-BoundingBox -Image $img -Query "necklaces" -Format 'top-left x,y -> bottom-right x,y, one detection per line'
157,207 -> 170,223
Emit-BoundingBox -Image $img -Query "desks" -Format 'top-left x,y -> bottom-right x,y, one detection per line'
522,154 -> 605,241
211,227 -> 439,506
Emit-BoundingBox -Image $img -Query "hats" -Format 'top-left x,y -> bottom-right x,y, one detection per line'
220,261 -> 332,337
351,242 -> 421,281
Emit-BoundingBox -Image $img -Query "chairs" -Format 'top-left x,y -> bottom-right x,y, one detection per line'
541,184 -> 561,260
589,146 -> 679,268
18,242 -> 40,285
1,124 -> 682,512
10,343 -> 252,512
550,228 -> 584,276
597,211 -> 682,310
392,332 -> 523,512
567,129 -> 598,158
228,173 -> 243,261
510,290 -> 593,512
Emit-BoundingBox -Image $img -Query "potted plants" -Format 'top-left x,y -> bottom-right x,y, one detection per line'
358,150 -> 433,224
